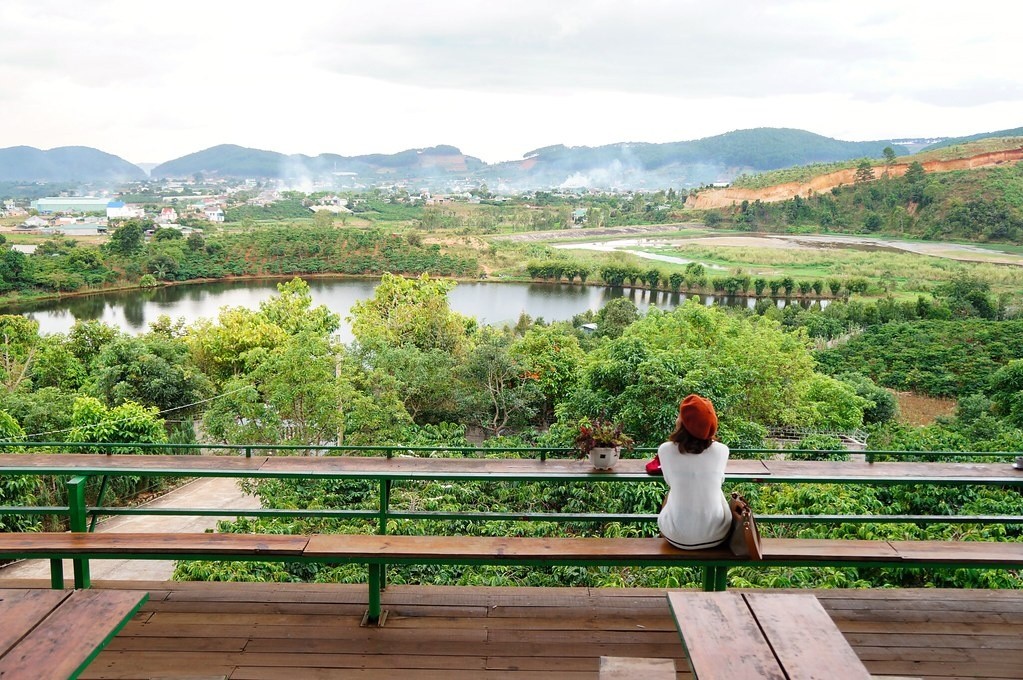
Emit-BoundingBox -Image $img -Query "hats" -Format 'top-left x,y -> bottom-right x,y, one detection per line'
680,395 -> 717,440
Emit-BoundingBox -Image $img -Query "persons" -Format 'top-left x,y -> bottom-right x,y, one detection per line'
657,393 -> 733,552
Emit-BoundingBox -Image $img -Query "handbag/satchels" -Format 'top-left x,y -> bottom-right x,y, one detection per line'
729,492 -> 763,562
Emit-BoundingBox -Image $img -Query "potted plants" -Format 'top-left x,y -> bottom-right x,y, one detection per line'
575,410 -> 635,470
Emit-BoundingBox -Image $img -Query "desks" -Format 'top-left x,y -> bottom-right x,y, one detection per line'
0,453 -> 1023,592
0,590 -> 148,680
666,592 -> 872,680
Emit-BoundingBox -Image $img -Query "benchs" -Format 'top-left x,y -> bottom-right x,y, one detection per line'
600,654 -> 675,680
0,532 -> 1023,630
